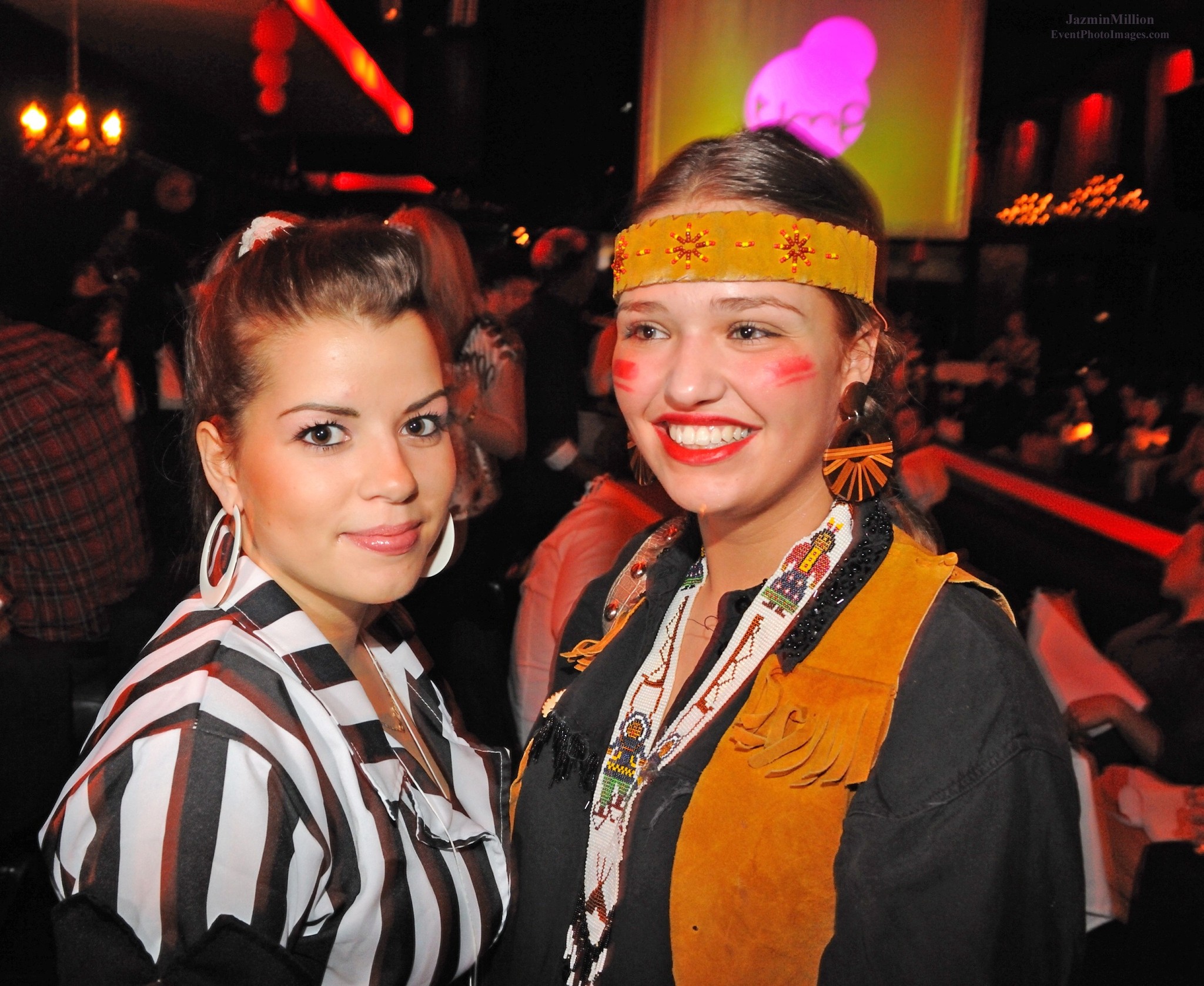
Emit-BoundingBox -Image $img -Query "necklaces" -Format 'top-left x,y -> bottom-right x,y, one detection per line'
373,656 -> 405,733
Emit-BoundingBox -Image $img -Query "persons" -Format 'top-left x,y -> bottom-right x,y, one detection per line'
0,126 -> 1204,986
